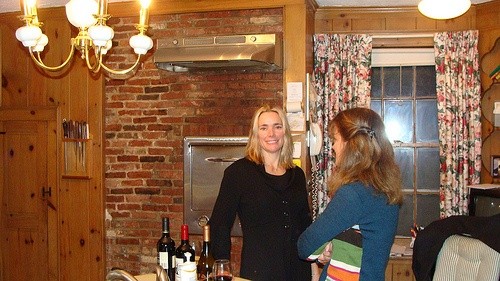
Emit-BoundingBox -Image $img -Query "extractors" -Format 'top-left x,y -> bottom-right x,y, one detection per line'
154,33 -> 283,74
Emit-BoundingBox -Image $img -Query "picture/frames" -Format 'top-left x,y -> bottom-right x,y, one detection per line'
491,155 -> 500,178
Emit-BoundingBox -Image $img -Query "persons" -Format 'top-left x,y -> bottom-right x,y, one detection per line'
198,105 -> 319,281
297,108 -> 403,281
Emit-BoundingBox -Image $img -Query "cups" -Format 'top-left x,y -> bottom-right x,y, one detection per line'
212,259 -> 233,281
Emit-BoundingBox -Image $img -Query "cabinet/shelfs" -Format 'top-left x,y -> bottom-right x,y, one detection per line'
385,252 -> 424,281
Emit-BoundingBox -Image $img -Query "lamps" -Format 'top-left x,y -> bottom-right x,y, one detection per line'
15,0 -> 153,80
418,0 -> 471,20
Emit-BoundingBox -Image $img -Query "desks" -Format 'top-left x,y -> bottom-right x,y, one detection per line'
135,272 -> 252,281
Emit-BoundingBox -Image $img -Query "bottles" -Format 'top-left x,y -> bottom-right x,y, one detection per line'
197,225 -> 218,281
174,225 -> 198,281
156,217 -> 176,281
